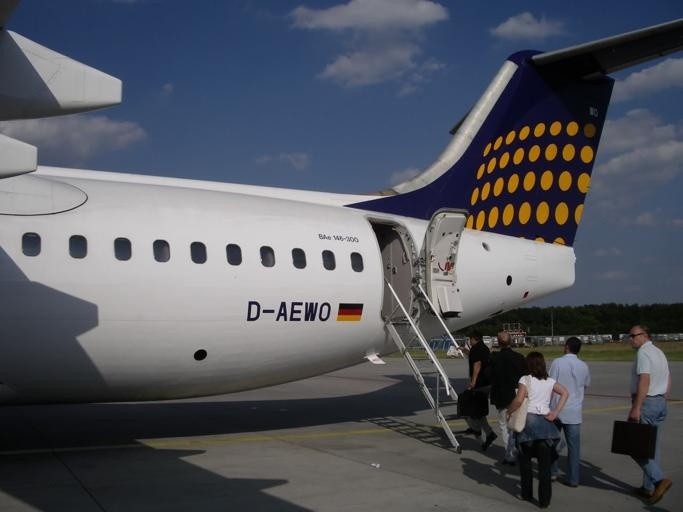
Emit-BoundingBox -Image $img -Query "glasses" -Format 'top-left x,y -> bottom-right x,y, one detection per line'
628,332 -> 646,338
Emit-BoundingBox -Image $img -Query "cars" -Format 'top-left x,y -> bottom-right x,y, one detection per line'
480,332 -> 682,345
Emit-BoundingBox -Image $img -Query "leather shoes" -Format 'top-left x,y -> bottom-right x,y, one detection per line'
643,478 -> 672,505
633,487 -> 655,497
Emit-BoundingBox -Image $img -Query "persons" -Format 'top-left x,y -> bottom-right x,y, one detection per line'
457,330 -> 497,451
627,324 -> 673,503
505,351 -> 570,508
482,332 -> 528,463
550,336 -> 591,487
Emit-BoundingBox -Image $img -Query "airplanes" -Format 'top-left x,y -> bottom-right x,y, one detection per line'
0,0 -> 683,456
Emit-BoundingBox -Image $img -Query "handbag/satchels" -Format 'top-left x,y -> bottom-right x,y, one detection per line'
503,374 -> 530,433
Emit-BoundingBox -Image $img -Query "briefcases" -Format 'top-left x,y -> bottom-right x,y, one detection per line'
457,388 -> 489,416
611,418 -> 657,459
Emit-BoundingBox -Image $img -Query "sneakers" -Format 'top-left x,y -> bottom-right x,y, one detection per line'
501,459 -> 516,468
549,475 -> 558,481
481,431 -> 497,450
